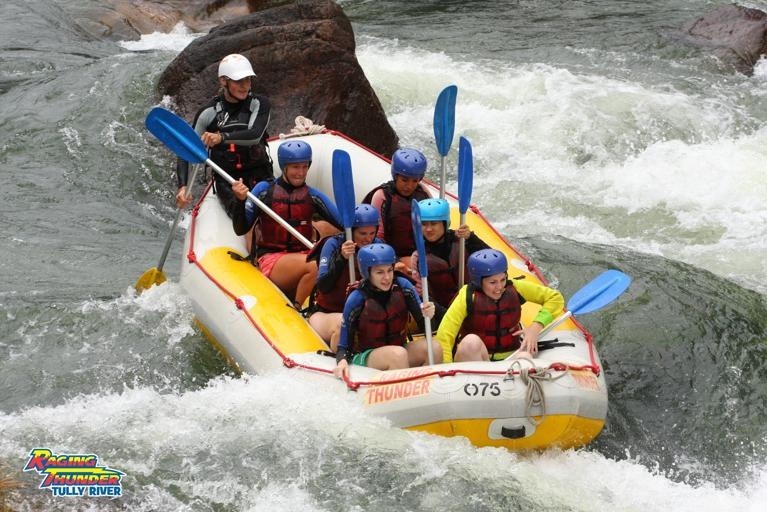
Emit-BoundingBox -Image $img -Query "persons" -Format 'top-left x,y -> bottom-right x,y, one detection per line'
334,243 -> 443,381
436,249 -> 565,363
307,203 -> 384,355
232,140 -> 345,311
411,198 -> 493,331
361,147 -> 434,269
175,53 -> 274,255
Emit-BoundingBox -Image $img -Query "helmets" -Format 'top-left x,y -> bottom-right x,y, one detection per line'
353,204 -> 380,230
278,141 -> 312,170
357,243 -> 397,280
391,148 -> 427,182
416,199 -> 451,235
217,53 -> 257,81
467,248 -> 507,290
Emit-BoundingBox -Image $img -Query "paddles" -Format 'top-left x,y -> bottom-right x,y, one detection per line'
508,270 -> 631,362
145,106 -> 317,249
332,149 -> 357,284
434,85 -> 457,197
411,199 -> 433,367
459,135 -> 473,289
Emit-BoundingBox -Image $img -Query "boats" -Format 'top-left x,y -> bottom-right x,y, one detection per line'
180,122 -> 610,455
